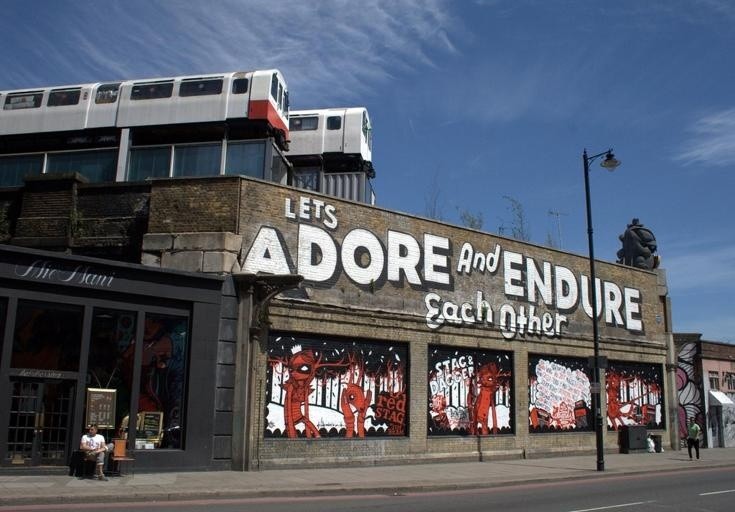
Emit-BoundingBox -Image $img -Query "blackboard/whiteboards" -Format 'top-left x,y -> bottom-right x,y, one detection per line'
140,411 -> 163,436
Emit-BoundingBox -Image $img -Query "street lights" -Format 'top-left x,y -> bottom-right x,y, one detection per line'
583,148 -> 620,471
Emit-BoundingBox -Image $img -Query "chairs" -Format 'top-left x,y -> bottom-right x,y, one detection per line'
77,440 -> 107,478
110,438 -> 136,477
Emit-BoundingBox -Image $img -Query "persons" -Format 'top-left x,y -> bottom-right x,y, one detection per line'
78,422 -> 115,482
686,416 -> 701,461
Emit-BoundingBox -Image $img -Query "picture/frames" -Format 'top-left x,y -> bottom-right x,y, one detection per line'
139,411 -> 163,444
84,388 -> 116,429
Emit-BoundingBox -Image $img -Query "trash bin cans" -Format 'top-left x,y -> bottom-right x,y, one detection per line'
650,435 -> 661,453
